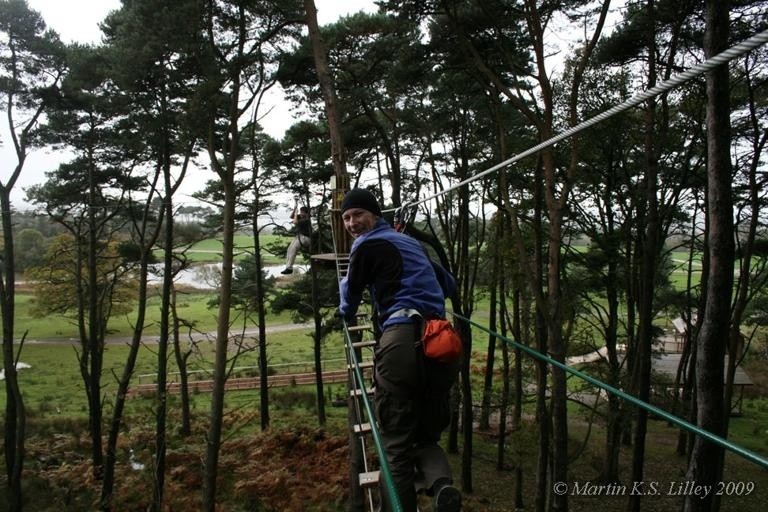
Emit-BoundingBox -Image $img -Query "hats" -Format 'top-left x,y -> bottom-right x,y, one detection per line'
339,189 -> 381,218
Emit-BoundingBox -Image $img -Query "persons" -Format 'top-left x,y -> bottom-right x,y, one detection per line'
280,205 -> 314,275
334,187 -> 465,512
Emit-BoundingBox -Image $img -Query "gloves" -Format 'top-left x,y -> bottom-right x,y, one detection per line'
334,307 -> 351,322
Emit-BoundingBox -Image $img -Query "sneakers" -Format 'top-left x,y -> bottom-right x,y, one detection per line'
281,269 -> 292,274
432,484 -> 461,512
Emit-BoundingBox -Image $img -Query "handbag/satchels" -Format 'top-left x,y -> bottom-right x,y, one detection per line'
421,318 -> 462,361
